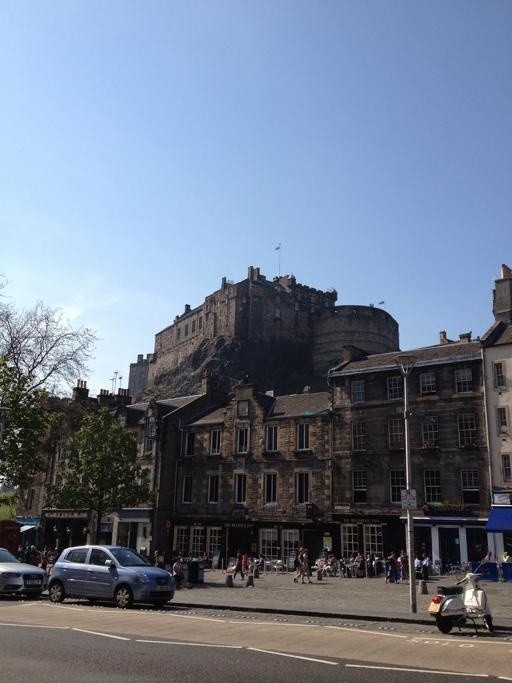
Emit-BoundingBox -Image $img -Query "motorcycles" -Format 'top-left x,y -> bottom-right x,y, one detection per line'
429,551 -> 495,635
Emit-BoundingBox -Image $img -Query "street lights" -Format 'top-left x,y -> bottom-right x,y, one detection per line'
391,353 -> 421,613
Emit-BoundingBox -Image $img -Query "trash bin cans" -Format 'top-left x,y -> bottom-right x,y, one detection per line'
159,560 -> 204,584
470,562 -> 512,582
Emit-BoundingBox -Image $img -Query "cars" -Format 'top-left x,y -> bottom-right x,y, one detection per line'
0,547 -> 48,598
47,545 -> 175,608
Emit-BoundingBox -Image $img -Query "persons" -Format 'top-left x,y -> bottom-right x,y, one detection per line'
151,547 -> 431,589
484,551 -> 492,560
503,551 -> 512,562
18,544 -> 48,569
